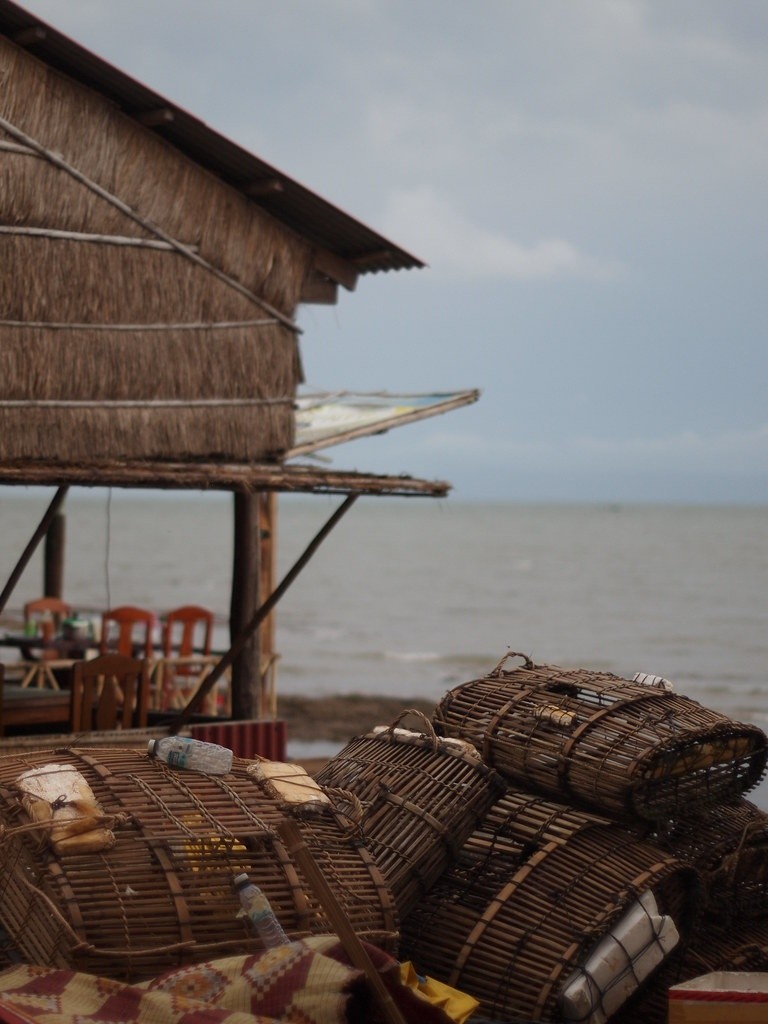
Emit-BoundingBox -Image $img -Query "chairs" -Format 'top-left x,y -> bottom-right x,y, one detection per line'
20,598 -> 215,731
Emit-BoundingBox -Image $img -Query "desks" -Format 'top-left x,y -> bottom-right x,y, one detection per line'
2,635 -> 100,660
1,685 -> 70,726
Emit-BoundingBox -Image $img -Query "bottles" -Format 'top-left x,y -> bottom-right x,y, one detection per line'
147,735 -> 234,777
231,872 -> 293,950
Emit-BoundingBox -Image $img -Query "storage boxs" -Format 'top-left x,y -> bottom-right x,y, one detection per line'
666,970 -> 768,1024
